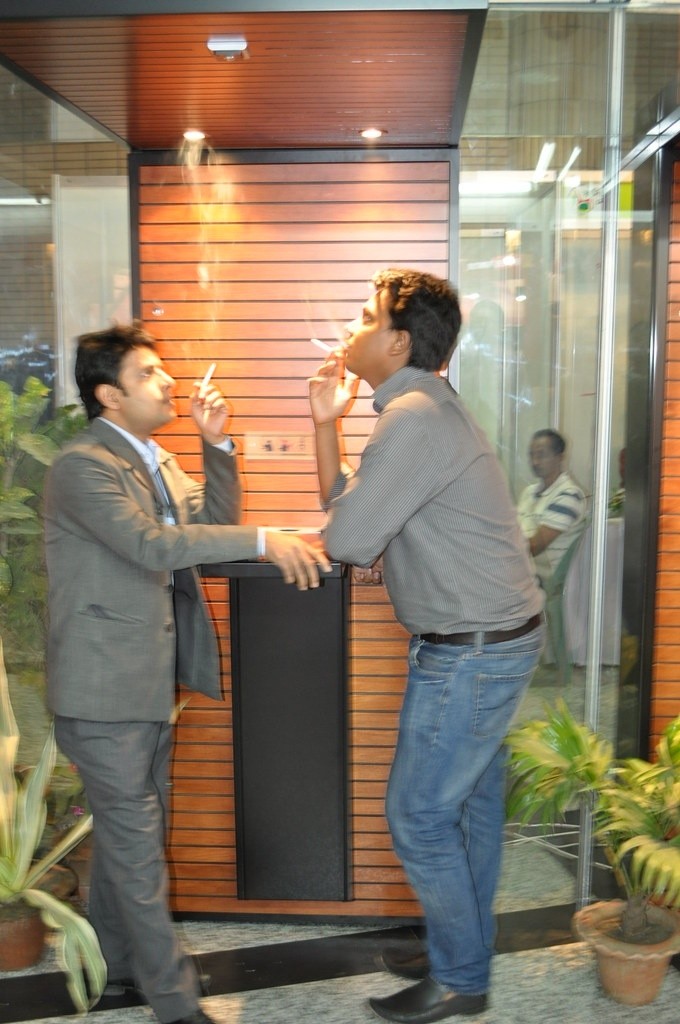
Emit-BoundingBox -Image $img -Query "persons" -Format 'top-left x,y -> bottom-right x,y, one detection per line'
46,326 -> 332,1024
609,449 -> 627,519
309,269 -> 547,1024
517,428 -> 587,597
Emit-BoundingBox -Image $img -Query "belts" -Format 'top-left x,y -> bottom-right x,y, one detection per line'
413,613 -> 541,645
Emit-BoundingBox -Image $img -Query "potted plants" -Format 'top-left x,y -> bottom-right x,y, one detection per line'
502,699 -> 679,1005
0,641 -> 107,1021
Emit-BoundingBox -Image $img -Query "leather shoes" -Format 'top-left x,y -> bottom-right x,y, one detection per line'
103,973 -> 212,995
162,1009 -> 215,1024
382,947 -> 430,979
369,977 -> 487,1024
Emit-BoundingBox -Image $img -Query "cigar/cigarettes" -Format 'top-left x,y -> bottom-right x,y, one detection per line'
197,362 -> 217,398
311,337 -> 332,352
